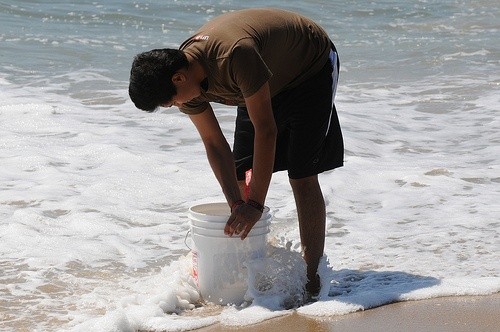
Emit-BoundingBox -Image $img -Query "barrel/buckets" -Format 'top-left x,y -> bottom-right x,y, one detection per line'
184,202 -> 271,305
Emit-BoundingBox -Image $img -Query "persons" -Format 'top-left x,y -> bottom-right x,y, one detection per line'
126,7 -> 345,311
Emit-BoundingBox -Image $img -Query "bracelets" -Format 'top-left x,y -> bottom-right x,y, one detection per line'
231,199 -> 245,214
246,198 -> 264,213
